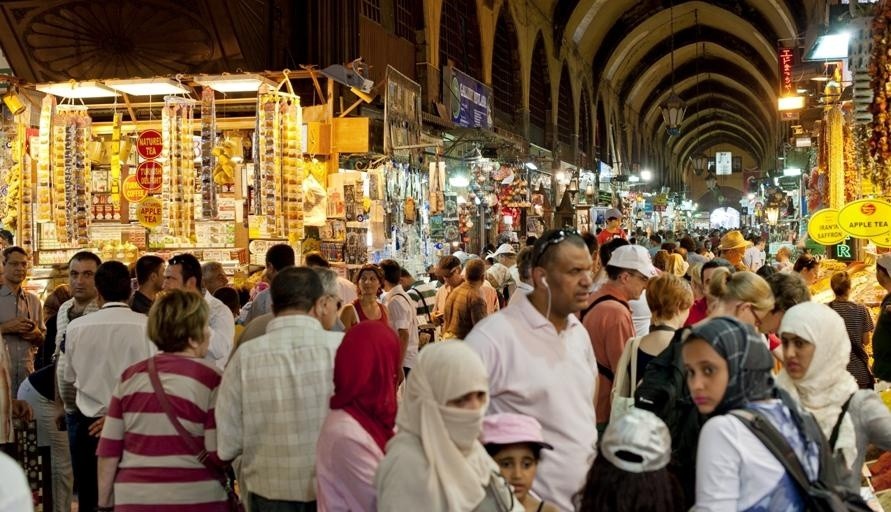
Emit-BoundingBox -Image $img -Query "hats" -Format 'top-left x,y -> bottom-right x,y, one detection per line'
716,231 -> 754,252
597,406 -> 677,477
606,244 -> 659,281
434,255 -> 461,277
492,242 -> 516,257
477,413 -> 555,452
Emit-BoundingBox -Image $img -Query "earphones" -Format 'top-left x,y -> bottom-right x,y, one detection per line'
541,276 -> 547,286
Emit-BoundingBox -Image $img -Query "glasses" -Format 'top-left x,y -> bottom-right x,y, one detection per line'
533,228 -> 581,268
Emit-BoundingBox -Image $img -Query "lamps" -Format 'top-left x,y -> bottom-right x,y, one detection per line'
704,105 -> 726,209
689,7 -> 707,176
656,0 -> 688,136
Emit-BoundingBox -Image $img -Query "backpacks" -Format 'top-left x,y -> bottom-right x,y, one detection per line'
631,326 -> 694,430
729,389 -> 877,512
